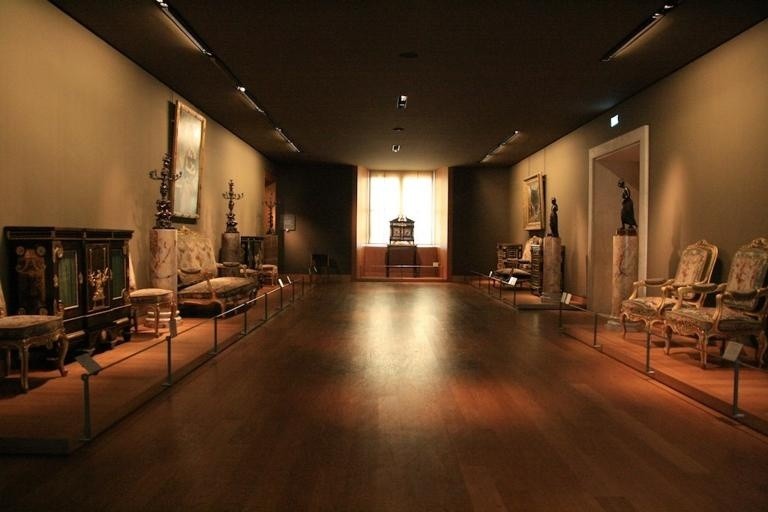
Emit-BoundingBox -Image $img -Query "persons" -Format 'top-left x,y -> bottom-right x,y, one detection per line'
549,197 -> 558,236
616,179 -> 638,229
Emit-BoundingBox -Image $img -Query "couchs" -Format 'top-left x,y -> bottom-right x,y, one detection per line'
176,226 -> 260,318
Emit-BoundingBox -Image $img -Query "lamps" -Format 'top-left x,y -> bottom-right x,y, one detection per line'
479,130 -> 519,165
155,0 -> 302,156
601,3 -> 673,62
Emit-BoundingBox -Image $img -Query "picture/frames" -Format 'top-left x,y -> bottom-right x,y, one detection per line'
171,100 -> 207,218
519,171 -> 545,231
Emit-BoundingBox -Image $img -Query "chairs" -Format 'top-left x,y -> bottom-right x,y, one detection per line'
664,238 -> 768,368
1,276 -> 72,390
308,254 -> 330,280
127,247 -> 176,339
385,212 -> 420,279
494,235 -> 543,306
620,238 -> 720,366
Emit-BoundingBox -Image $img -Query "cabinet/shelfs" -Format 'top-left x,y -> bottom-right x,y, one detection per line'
263,234 -> 281,268
2,226 -> 140,353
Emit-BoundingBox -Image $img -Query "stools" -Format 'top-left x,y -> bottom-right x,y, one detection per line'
261,264 -> 279,287
